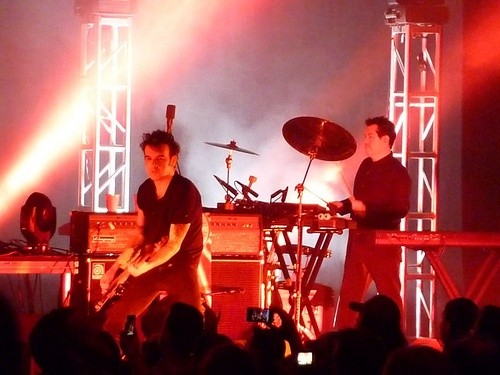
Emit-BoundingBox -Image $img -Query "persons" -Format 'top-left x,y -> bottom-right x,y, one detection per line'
0,294 -> 500,375
98,129 -> 204,357
328,116 -> 414,338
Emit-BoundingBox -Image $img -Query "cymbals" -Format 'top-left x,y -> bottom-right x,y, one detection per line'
205,141 -> 259,156
282,116 -> 357,161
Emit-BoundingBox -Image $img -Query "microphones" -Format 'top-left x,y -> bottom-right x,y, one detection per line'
236,181 -> 258,197
213,175 -> 238,196
165,105 -> 176,135
281,187 -> 289,203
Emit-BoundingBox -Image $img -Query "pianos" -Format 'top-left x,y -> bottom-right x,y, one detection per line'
374,228 -> 500,304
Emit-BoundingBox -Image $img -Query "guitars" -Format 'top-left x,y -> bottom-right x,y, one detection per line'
95,235 -> 168,312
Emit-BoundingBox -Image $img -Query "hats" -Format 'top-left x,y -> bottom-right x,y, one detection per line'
349,296 -> 400,320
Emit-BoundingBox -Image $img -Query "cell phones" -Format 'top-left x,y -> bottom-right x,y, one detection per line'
125,314 -> 138,338
296,350 -> 314,365
247,307 -> 273,323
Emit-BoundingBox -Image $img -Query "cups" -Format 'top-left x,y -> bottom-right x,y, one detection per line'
105,194 -> 119,212
